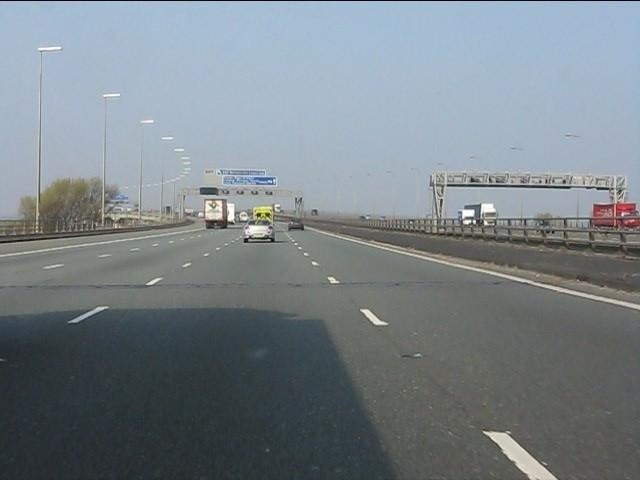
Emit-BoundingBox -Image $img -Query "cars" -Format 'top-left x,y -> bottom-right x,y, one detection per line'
242,218 -> 275,243
288,219 -> 304,231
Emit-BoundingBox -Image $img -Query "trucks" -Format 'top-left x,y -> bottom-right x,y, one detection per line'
204,198 -> 227,229
458,208 -> 477,226
463,202 -> 497,228
592,202 -> 640,231
227,202 -> 235,224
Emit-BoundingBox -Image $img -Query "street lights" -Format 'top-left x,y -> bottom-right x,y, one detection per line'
101,92 -> 121,227
138,119 -> 155,219
35,45 -> 63,233
